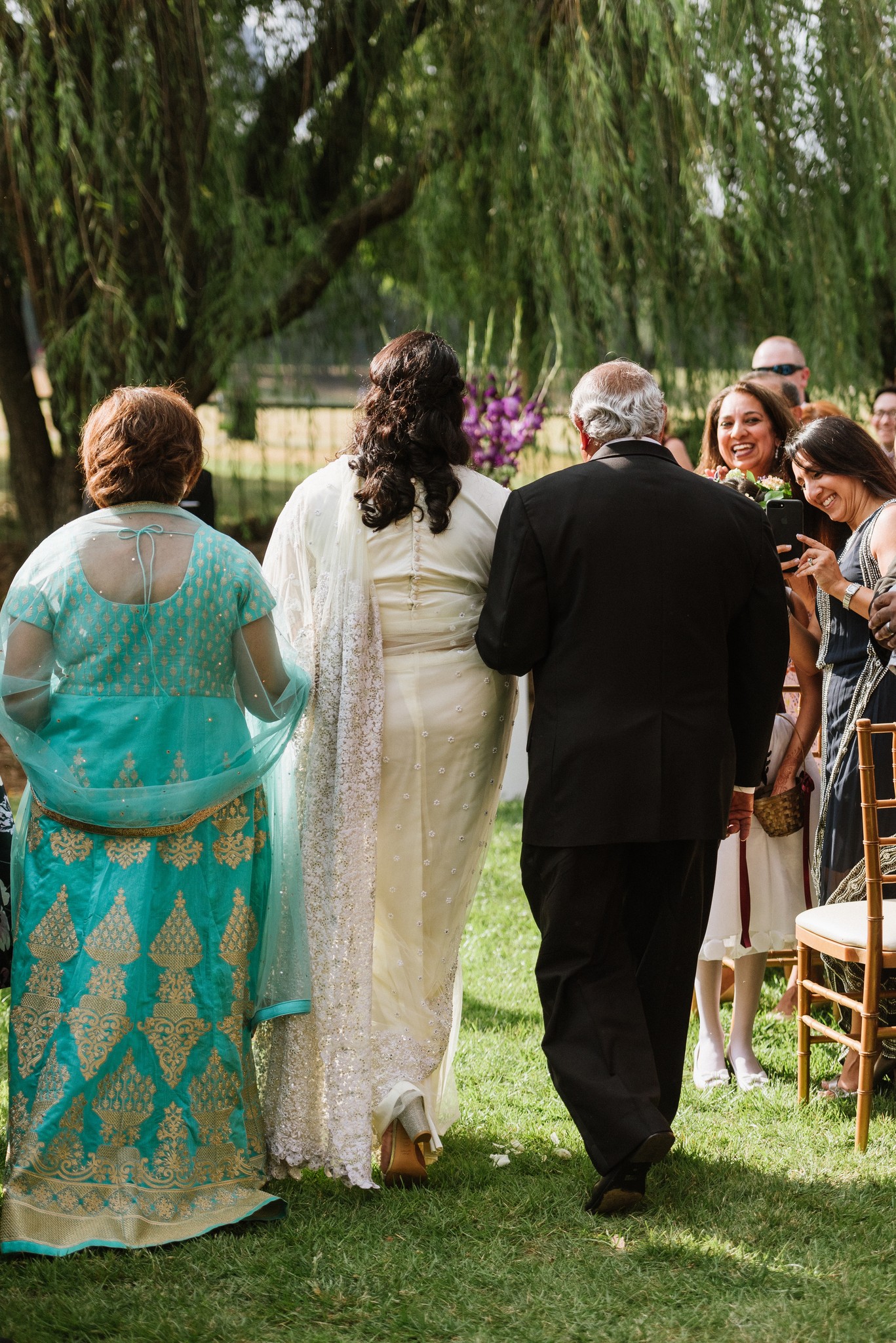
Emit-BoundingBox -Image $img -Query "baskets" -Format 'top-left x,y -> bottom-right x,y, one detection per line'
752,711 -> 816,840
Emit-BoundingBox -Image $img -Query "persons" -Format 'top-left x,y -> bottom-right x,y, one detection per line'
866,552 -> 896,653
751,335 -> 813,408
739,369 -> 803,427
0,376 -> 316,1264
473,359 -> 796,1217
869,387 -> 896,468
769,412 -> 896,1104
246,327 -> 518,1202
689,378 -> 824,1093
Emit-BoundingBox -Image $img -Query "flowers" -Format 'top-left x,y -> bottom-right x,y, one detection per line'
439,296 -> 566,489
704,463 -> 793,518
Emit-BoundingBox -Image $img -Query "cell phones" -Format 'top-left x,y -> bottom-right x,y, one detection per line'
766,498 -> 805,572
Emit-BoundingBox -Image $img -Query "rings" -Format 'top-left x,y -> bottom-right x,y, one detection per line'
807,557 -> 813,566
886,621 -> 894,636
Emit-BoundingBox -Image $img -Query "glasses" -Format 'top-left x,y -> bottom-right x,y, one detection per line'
750,362 -> 803,378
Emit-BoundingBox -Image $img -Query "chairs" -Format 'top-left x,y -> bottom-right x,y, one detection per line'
788,721 -> 896,1157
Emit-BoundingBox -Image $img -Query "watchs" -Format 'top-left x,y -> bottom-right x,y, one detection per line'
842,582 -> 864,611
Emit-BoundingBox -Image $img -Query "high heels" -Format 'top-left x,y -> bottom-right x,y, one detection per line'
379,1089 -> 432,1190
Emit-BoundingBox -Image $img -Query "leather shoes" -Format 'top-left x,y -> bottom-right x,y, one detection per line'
582,1129 -> 676,1219
692,1040 -> 730,1091
726,1041 -> 770,1091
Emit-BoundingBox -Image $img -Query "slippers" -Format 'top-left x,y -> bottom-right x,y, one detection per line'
816,1076 -> 859,1099
